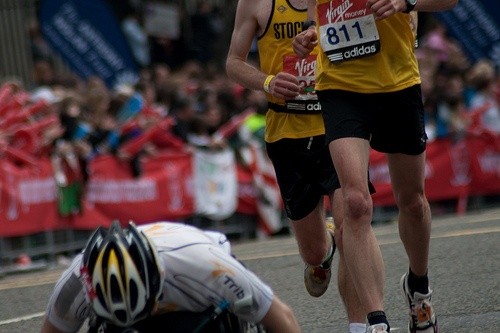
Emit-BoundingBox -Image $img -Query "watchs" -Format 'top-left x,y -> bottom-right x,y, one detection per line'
402,0 -> 417,14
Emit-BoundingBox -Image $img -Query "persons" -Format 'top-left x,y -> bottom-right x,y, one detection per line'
226,0 -> 376,333
41,220 -> 302,333
14,19 -> 500,267
293,0 -> 460,333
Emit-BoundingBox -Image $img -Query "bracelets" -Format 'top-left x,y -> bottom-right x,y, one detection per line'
302,20 -> 317,32
264,75 -> 275,93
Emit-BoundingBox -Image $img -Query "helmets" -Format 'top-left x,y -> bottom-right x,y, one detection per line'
80,219 -> 166,327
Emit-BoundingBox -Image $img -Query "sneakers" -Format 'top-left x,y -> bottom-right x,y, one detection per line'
304,216 -> 337,296
401,271 -> 441,333
365,322 -> 391,333
